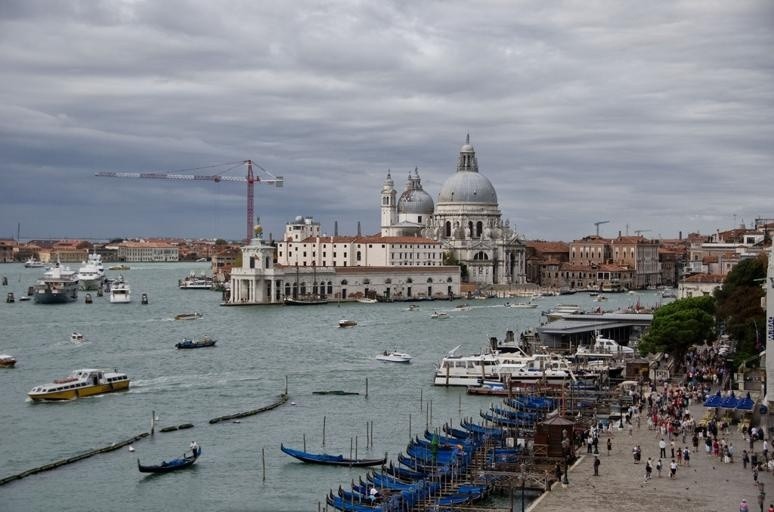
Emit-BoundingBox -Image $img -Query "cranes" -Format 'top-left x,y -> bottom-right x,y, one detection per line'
92,159 -> 284,243
635,229 -> 652,236
594,221 -> 610,236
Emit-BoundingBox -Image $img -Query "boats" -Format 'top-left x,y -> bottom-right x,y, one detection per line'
0,353 -> 14,368
71,330 -> 82,342
402,285 -> 680,325
282,292 -> 330,306
357,296 -> 379,305
174,334 -> 216,349
181,272 -> 212,290
136,441 -> 200,475
28,367 -> 129,404
0,243 -> 149,305
174,311 -> 200,321
279,442 -> 387,468
336,318 -> 356,326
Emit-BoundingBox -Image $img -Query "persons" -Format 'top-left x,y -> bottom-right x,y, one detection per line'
368,486 -> 380,503
768,504 -> 774,512
554,346 -> 774,492
756,490 -> 766,512
738,498 -> 749,512
188,440 -> 197,458
